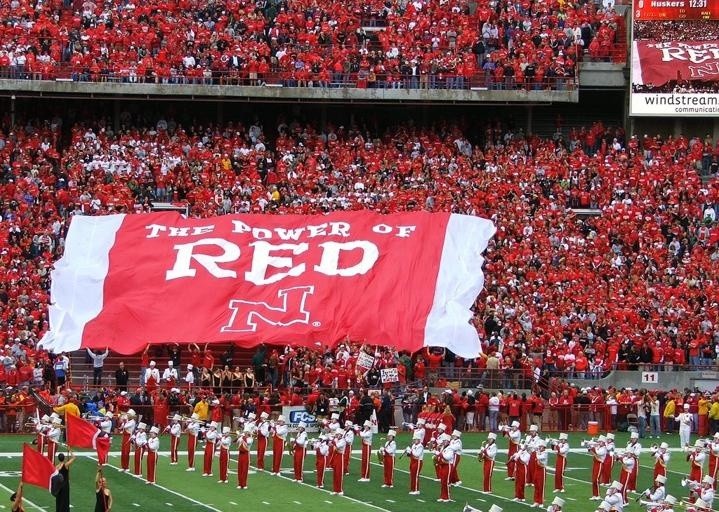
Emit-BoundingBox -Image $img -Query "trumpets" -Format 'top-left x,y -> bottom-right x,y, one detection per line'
581,438 -> 599,447
651,443 -> 661,453
496,421 -> 514,431
544,435 -> 562,443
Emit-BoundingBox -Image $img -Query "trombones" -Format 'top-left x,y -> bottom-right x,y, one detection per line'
24,414 -> 104,430
166,415 -> 206,426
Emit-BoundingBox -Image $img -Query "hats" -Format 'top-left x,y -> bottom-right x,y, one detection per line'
489,503 -> 503,512
598,500 -> 611,512
36,409 -> 719,454
702,475 -> 716,486
119,361 -> 266,396
664,494 -> 677,506
655,474 -> 669,485
694,498 -> 709,510
683,403 -> 690,409
444,383 -> 719,400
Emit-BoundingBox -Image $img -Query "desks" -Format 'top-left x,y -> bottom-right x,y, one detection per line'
150,201 -> 190,220
572,208 -> 603,217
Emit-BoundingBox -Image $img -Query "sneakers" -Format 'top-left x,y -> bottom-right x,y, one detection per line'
117,459 -> 636,508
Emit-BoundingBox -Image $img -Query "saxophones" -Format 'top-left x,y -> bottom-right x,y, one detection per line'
478,440 -> 487,463
376,437 -> 386,465
428,431 -> 436,452
